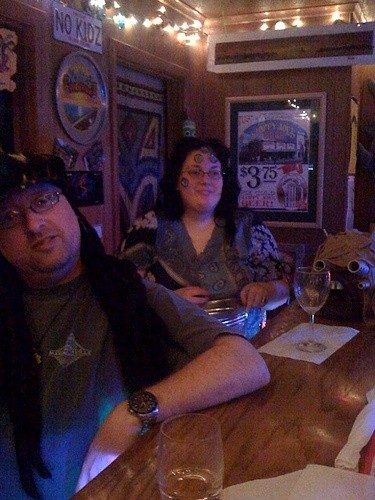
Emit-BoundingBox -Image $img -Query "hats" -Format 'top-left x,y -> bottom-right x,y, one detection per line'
0,152 -> 68,202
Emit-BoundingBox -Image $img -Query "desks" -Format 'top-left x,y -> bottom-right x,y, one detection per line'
71,298 -> 375,500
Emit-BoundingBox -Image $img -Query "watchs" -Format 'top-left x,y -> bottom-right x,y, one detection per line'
127,391 -> 159,436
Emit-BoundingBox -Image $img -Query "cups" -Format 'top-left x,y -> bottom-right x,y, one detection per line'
158,413 -> 224,500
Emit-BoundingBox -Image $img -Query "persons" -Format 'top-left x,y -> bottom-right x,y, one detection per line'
119,138 -> 293,328
0,152 -> 270,500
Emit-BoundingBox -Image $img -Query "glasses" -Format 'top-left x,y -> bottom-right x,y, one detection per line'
182,167 -> 225,180
0,192 -> 64,230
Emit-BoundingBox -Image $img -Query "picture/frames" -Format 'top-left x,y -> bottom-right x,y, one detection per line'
0,0 -> 47,154
108,37 -> 192,258
224,92 -> 327,228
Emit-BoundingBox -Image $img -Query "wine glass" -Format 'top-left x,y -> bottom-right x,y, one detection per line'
293,266 -> 331,352
